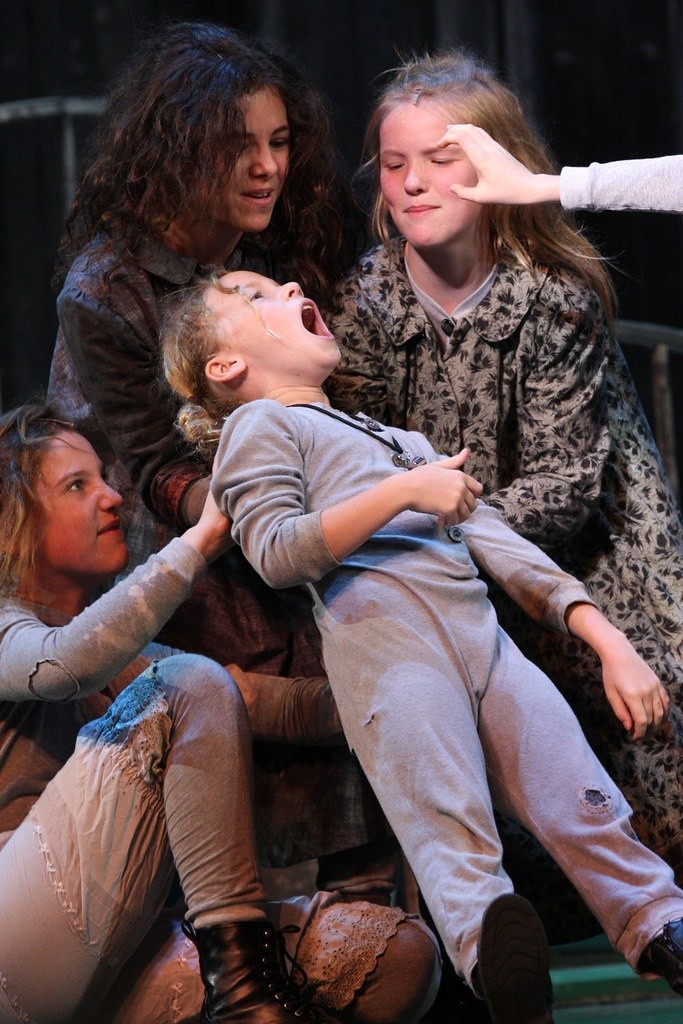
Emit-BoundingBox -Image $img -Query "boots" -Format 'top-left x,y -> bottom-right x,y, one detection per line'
181,918 -> 332,1024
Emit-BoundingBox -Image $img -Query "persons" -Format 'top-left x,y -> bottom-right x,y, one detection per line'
437,122 -> 683,215
314,48 -> 683,891
53,23 -> 405,906
0,403 -> 440,1024
158,269 -> 683,1024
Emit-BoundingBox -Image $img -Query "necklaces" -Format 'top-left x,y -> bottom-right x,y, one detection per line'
288,403 -> 424,470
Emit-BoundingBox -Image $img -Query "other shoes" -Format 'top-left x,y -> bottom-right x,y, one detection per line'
647,918 -> 683,993
475,894 -> 552,1024
